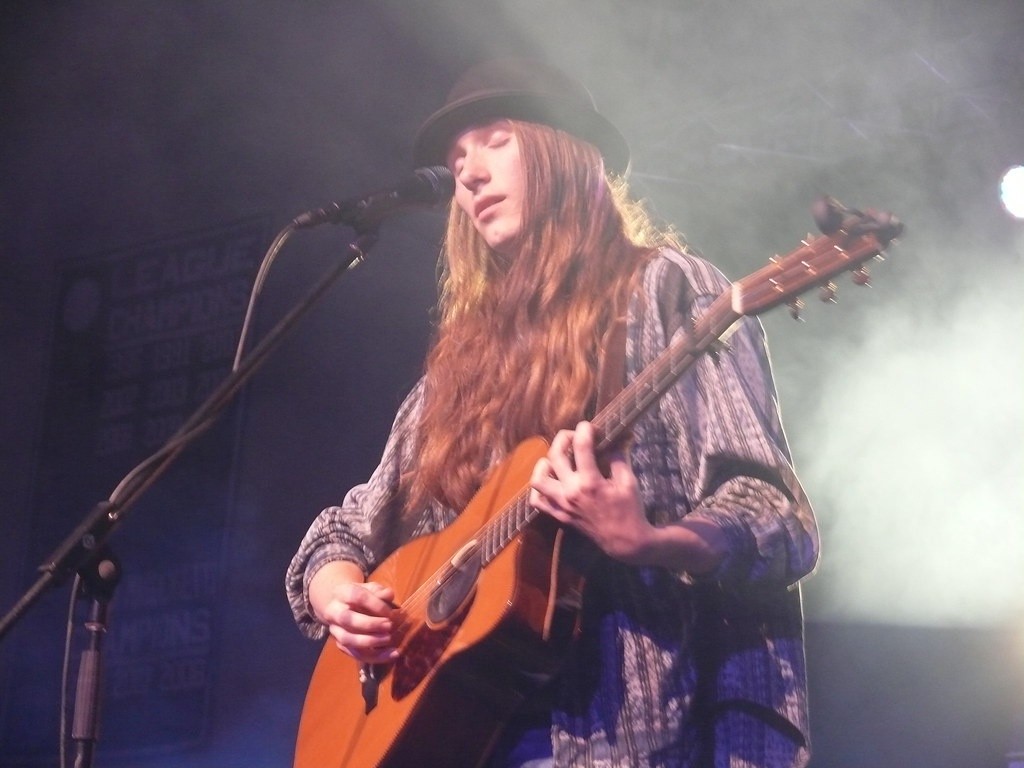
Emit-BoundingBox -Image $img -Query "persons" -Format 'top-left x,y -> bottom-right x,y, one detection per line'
285,57 -> 820,768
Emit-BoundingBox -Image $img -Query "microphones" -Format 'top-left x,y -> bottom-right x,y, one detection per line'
292,165 -> 456,232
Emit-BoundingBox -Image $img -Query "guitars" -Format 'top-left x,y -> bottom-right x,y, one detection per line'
292,210 -> 902,768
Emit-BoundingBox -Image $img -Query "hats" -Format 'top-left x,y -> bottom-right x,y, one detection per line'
413,56 -> 631,181
